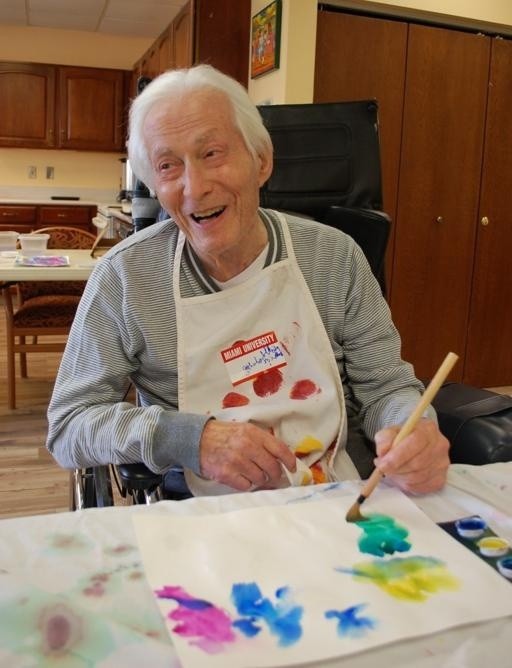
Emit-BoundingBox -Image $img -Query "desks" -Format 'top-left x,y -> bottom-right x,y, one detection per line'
1,460 -> 512,668
0,247 -> 106,288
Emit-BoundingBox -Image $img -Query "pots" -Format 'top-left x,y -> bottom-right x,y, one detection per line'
117,157 -> 150,199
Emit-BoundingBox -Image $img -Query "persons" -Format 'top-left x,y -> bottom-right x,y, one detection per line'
257,28 -> 266,63
42,64 -> 451,496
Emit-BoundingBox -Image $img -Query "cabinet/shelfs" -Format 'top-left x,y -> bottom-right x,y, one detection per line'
314,1 -> 409,307
1,60 -> 132,155
388,9 -> 512,388
133,0 -> 252,103
0,204 -> 99,245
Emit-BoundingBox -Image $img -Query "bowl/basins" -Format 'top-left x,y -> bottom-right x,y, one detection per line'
0,231 -> 51,257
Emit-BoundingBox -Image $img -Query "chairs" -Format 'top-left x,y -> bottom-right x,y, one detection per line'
1,225 -> 102,410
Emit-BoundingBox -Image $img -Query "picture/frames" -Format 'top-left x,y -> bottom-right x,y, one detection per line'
247,0 -> 282,78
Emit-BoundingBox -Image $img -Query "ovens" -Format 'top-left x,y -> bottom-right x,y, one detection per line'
92,213 -> 114,239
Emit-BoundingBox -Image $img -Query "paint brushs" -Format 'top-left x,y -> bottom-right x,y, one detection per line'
345,352 -> 458,522
